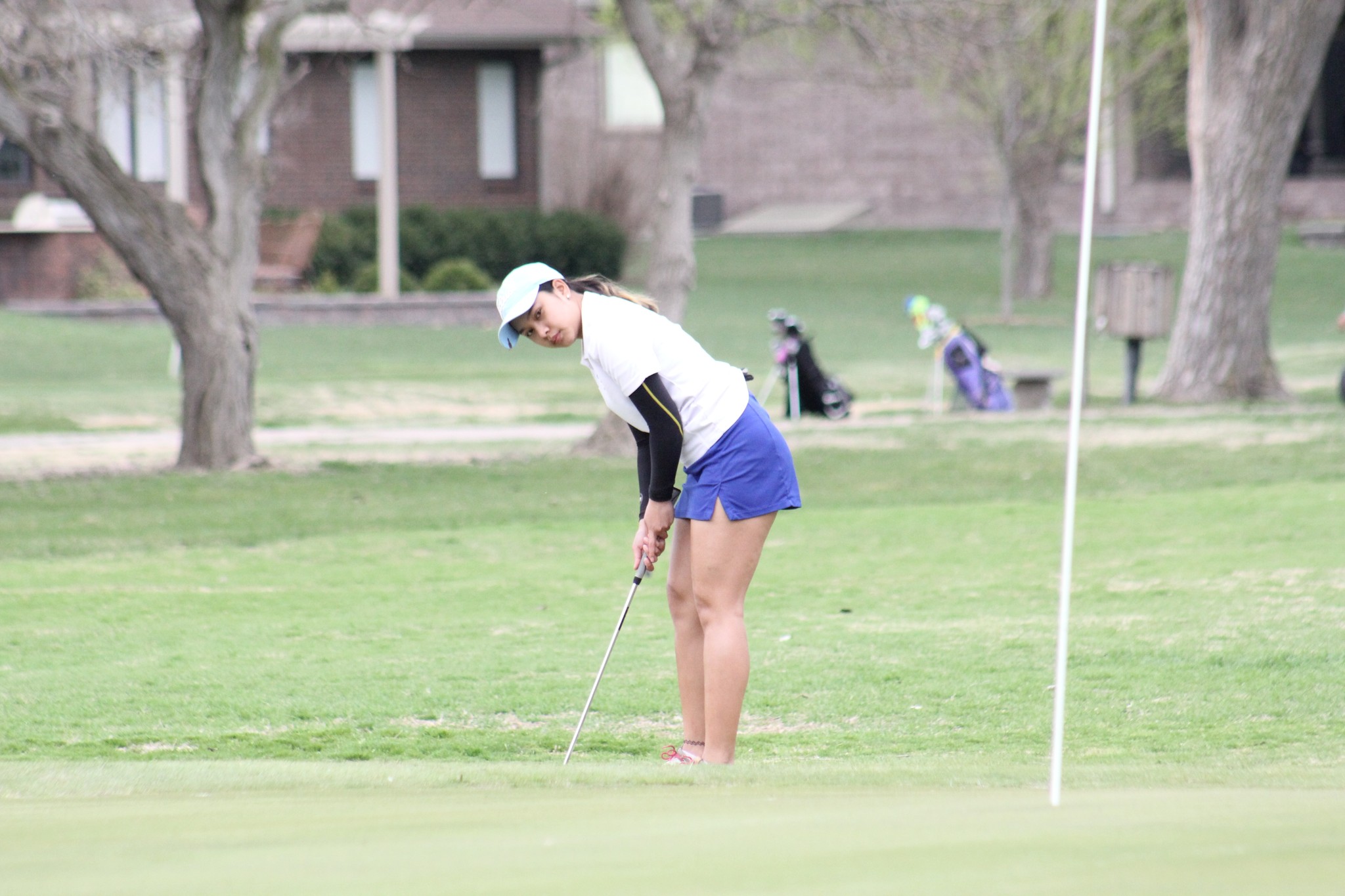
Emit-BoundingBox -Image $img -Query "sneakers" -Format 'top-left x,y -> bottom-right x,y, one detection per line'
660,745 -> 699,765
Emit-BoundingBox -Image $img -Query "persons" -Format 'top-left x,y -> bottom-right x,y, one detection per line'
498,262 -> 804,765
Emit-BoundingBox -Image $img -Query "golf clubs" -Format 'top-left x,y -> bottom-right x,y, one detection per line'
562,486 -> 683,766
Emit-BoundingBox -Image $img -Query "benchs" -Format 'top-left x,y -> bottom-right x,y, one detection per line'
980,367 -> 1061,411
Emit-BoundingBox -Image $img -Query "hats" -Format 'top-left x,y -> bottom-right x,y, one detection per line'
496,261 -> 565,348
